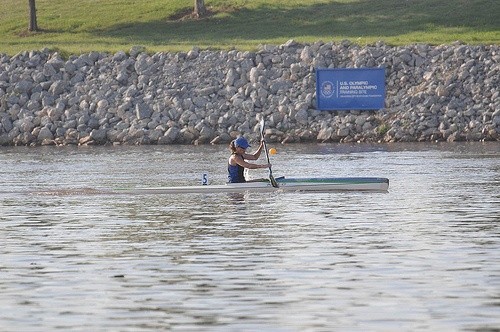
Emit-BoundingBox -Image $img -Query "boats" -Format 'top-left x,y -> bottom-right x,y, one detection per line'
111,176 -> 391,194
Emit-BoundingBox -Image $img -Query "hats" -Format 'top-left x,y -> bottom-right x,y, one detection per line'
234,137 -> 251,149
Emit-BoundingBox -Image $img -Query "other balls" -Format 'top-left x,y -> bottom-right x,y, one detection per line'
269,148 -> 276,154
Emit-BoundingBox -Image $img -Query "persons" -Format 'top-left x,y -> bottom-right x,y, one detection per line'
228,137 -> 286,183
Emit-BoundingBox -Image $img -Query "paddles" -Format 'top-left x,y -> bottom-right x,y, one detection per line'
258,120 -> 279,188
